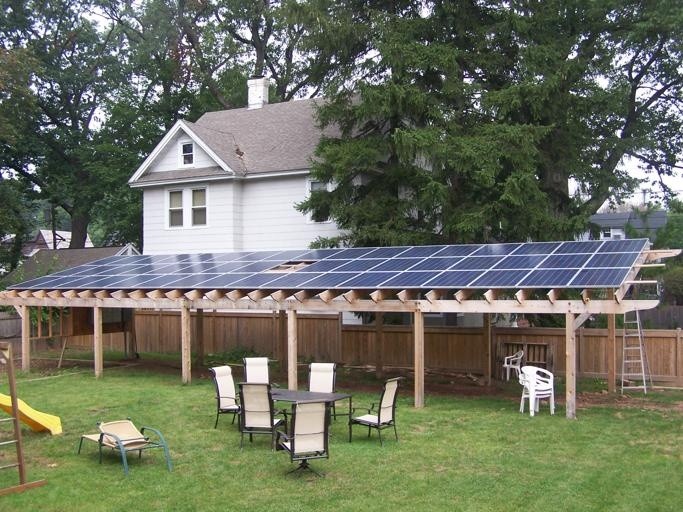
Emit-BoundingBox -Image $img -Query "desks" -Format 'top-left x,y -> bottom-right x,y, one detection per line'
271,388 -> 353,443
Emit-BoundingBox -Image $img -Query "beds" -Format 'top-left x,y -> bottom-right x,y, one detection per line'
77,416 -> 173,477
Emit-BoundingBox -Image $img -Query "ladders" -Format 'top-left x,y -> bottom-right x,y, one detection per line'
621,312 -> 655,396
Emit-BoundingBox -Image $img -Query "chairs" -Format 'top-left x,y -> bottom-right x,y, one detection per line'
518,373 -> 555,414
521,365 -> 554,411
236,381 -> 287,453
348,375 -> 404,447
501,350 -> 524,381
275,400 -> 331,479
307,362 -> 338,420
519,380 -> 555,417
242,356 -> 271,384
207,365 -> 241,432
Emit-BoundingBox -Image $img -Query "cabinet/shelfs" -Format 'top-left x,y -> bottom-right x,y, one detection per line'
495,342 -> 552,380
